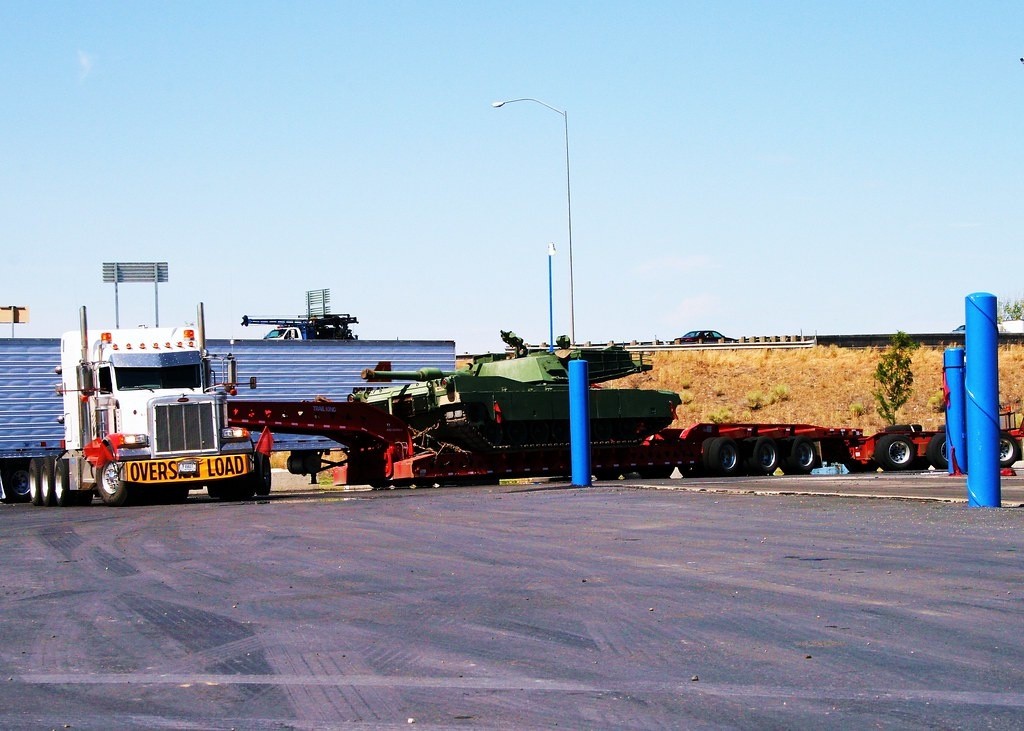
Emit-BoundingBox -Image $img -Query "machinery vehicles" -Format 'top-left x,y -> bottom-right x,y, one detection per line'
347,329 -> 681,457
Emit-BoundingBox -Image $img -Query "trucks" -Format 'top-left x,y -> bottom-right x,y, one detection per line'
241,313 -> 360,340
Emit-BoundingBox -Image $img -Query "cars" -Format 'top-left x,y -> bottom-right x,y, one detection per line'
674,330 -> 735,345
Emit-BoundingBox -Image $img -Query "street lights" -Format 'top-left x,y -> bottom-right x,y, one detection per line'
490,98 -> 576,349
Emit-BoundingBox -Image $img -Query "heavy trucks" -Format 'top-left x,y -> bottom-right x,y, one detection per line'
28,300 -> 272,506
